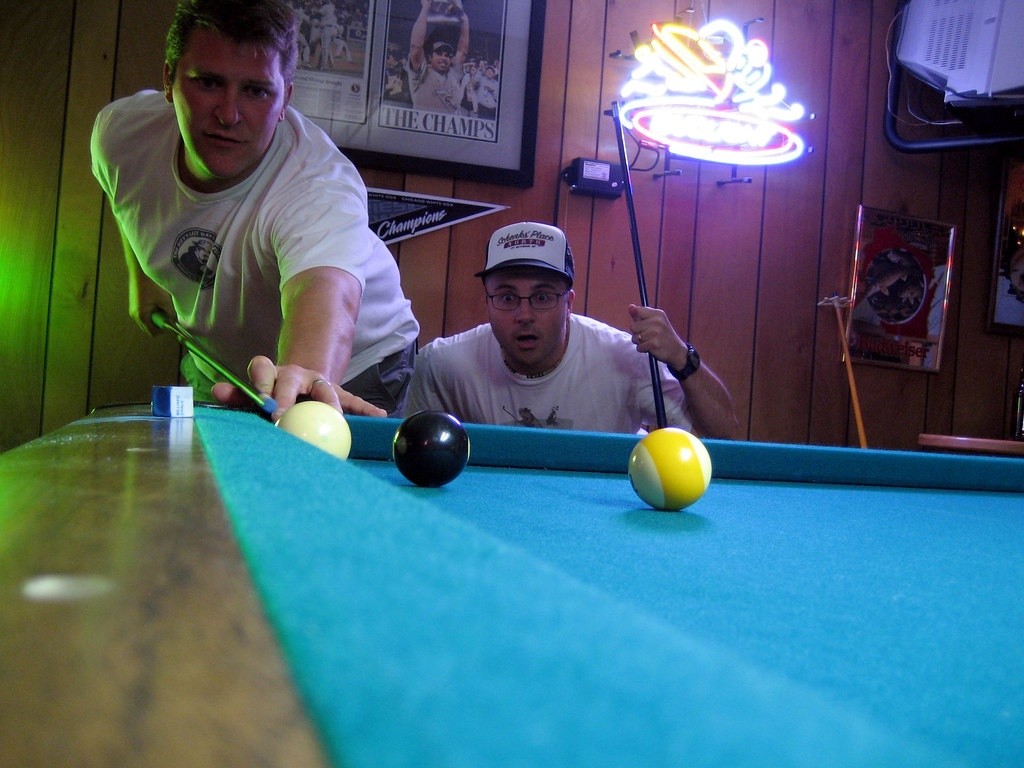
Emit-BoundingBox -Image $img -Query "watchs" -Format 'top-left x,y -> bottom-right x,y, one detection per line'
666,342 -> 701,382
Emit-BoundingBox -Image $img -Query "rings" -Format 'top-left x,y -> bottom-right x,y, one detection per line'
306,380 -> 331,396
638,333 -> 642,341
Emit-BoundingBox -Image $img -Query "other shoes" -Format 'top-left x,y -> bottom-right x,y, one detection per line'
319,65 -> 336,72
302,63 -> 312,69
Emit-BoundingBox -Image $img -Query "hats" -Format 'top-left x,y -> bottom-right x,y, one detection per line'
473,222 -> 574,283
430,38 -> 453,57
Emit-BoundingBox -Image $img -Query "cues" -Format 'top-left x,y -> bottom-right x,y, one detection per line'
609,97 -> 670,430
148,305 -> 279,415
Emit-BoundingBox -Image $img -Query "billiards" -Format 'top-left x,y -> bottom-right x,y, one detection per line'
391,408 -> 474,489
274,398 -> 353,464
626,426 -> 717,512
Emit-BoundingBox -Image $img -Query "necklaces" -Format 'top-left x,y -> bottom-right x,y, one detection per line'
499,319 -> 570,379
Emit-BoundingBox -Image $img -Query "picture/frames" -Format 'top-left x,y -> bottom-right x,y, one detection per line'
294,0 -> 548,188
985,150 -> 1023,340
841,202 -> 960,377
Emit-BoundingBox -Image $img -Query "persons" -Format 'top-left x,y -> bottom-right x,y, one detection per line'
90,0 -> 421,417
401,222 -> 746,439
291,1 -> 352,72
384,0 -> 499,121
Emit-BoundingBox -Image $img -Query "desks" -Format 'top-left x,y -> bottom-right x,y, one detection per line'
0,405 -> 1024,768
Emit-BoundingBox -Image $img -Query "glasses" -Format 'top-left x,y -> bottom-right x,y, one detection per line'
482,279 -> 570,311
